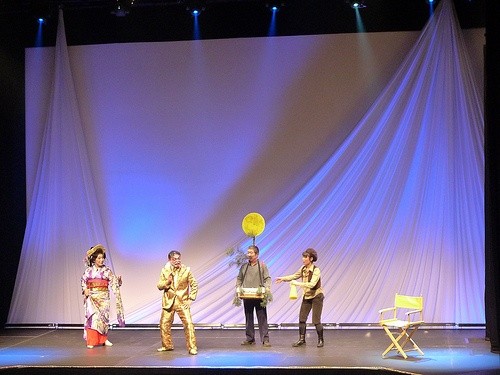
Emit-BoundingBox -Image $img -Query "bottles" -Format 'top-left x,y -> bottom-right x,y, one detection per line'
288,280 -> 297,299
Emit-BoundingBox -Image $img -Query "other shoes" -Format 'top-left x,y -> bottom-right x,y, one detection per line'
101,340 -> 112,346
86,344 -> 94,349
157,346 -> 174,351
262,341 -> 272,347
189,349 -> 198,354
241,340 -> 256,345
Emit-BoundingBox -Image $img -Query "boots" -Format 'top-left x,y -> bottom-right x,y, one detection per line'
295,333 -> 305,347
316,329 -> 324,347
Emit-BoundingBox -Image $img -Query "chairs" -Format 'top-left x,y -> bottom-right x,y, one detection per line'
378,293 -> 425,360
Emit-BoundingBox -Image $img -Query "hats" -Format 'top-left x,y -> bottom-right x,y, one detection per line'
86,244 -> 106,261
306,248 -> 318,261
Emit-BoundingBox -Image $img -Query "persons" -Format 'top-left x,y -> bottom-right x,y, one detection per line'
80,244 -> 125,349
235,245 -> 271,347
158,251 -> 198,355
276,248 -> 325,347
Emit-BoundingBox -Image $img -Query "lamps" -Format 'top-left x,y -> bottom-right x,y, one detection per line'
32,13 -> 48,24
184,0 -> 208,16
110,0 -> 131,17
